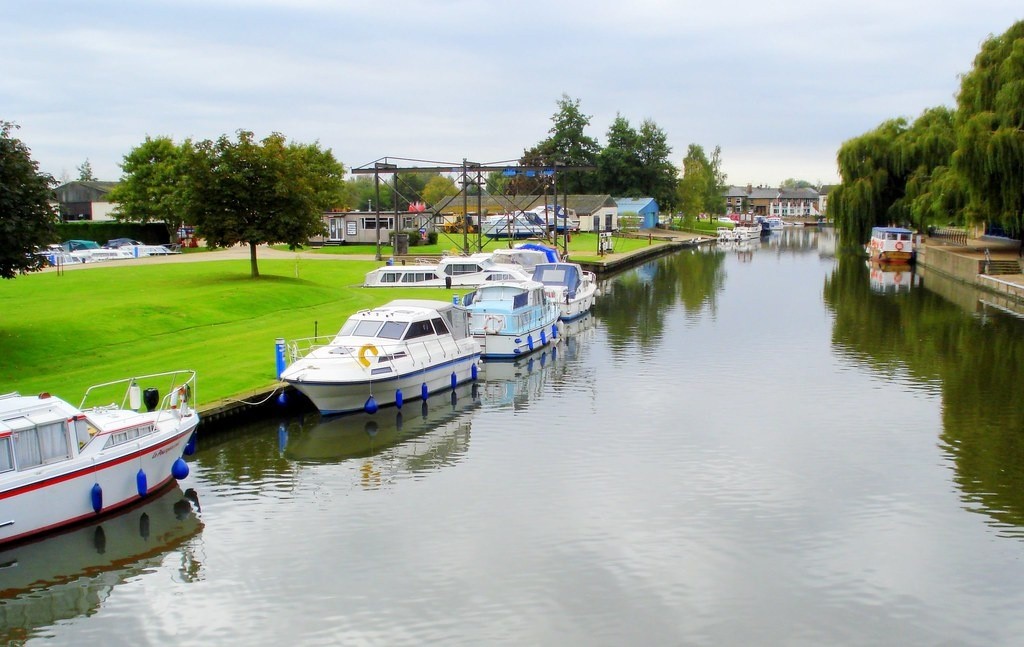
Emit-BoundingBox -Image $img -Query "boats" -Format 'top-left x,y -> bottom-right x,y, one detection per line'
864,226 -> 914,262
453,278 -> 563,356
717,215 -> 818,241
1,480 -> 205,603
276,299 -> 485,414
277,376 -> 482,464
0,369 -> 200,544
558,311 -> 596,338
476,335 -> 566,384
535,263 -> 598,320
362,243 -> 571,287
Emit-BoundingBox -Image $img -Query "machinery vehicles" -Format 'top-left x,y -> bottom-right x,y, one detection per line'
443,213 -> 475,234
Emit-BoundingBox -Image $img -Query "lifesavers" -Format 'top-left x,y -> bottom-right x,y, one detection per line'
483,314 -> 503,335
169,385 -> 189,422
357,342 -> 379,368
895,241 -> 904,250
